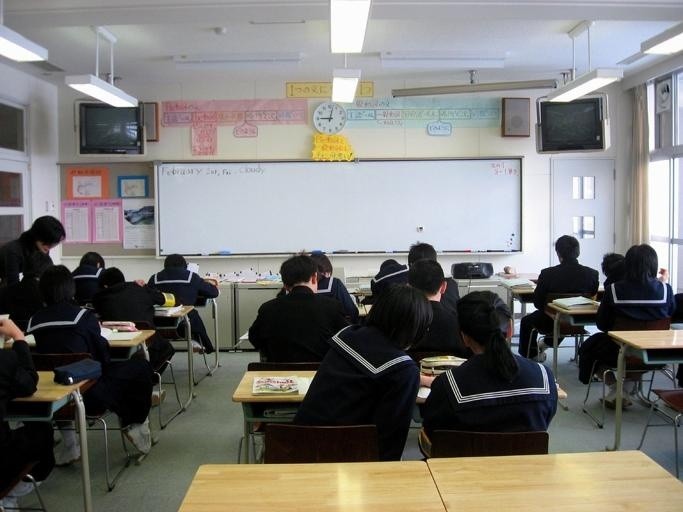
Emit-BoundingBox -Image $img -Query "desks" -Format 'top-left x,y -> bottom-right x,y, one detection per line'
503,275 -> 682,479
176,459 -> 446,512
424,449 -> 682,512
1,284 -> 219,511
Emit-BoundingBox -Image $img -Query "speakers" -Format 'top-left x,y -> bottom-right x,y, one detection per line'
502,97 -> 531,137
142,101 -> 160,142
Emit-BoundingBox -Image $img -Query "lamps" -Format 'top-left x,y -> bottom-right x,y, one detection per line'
331,55 -> 361,103
392,71 -> 558,98
0,2 -> 48,65
330,0 -> 372,54
641,21 -> 683,56
66,23 -> 139,108
546,19 -> 623,103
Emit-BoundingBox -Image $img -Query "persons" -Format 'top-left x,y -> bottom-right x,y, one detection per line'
1,215 -> 219,510
248,243 -> 558,463
515,234 -> 683,410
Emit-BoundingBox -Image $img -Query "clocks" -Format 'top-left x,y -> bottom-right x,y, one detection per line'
312,101 -> 347,137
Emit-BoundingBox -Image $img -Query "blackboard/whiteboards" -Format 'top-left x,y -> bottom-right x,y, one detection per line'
153,155 -> 524,259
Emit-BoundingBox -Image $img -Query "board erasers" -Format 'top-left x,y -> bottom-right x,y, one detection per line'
219,250 -> 229,256
313,250 -> 322,253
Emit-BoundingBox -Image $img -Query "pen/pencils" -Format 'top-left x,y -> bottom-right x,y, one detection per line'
432,366 -> 435,377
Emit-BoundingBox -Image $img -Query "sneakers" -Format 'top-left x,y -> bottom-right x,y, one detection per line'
599,393 -> 633,408
0,481 -> 41,512
123,425 -> 151,454
54,438 -> 81,464
534,340 -> 547,362
152,389 -> 166,406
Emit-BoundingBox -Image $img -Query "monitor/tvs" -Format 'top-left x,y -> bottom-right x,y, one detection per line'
541,98 -> 603,151
77,103 -> 144,155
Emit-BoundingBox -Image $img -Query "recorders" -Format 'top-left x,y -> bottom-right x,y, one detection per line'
452,262 -> 493,278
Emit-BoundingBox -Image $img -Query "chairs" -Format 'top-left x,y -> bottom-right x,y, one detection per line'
233,290 -> 567,461
